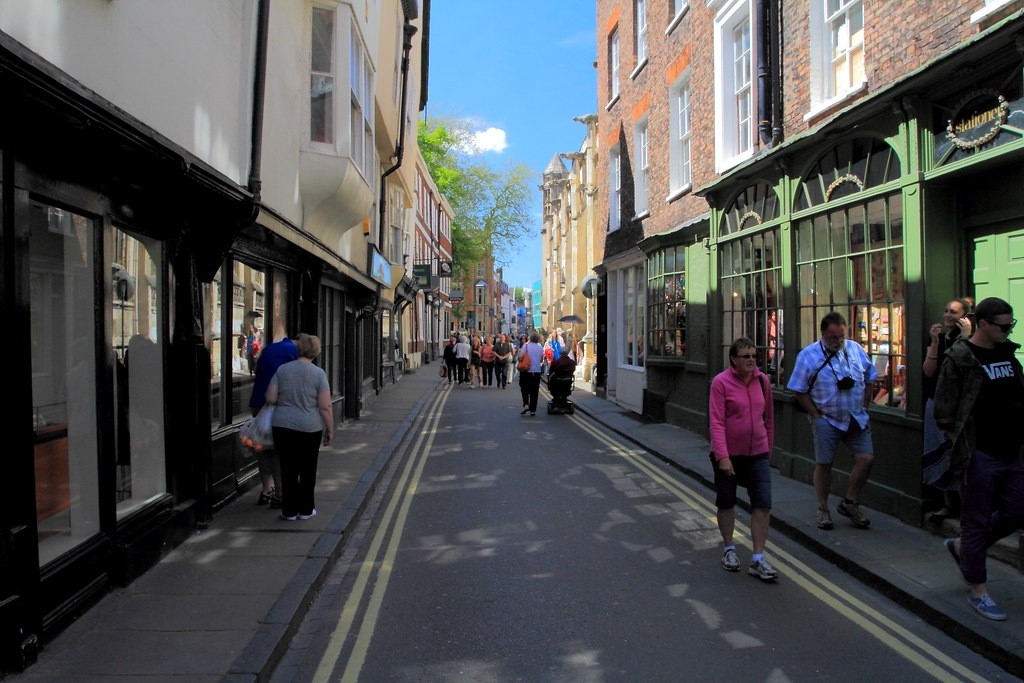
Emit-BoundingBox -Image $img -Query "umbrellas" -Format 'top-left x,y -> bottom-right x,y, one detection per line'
556,314 -> 585,323
245,310 -> 263,318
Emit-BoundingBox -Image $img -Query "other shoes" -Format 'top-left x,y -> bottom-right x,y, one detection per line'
298,509 -> 316,519
530,411 -> 537,415
519,406 -> 531,415
479,382 -> 484,388
449,382 -> 452,384
458,383 -> 463,386
469,384 -> 476,389
928,513 -> 950,527
279,511 -> 299,520
455,380 -> 459,383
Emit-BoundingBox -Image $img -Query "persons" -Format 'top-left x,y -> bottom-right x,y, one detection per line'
438,333 -> 527,389
923,297 -> 974,523
709,338 -> 779,578
238,326 -> 263,375
550,347 -> 576,380
544,328 -> 573,367
251,334 -> 334,521
785,312 -> 878,531
934,296 -> 1024,620
518,332 -> 544,415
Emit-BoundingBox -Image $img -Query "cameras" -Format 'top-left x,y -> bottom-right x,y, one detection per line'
837,376 -> 855,390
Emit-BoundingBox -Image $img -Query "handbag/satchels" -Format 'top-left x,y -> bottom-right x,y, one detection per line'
516,344 -> 532,372
242,403 -> 278,452
512,355 -> 517,364
792,394 -> 816,414
439,364 -> 449,378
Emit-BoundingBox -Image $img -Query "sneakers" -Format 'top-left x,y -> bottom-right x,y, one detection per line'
816,505 -> 835,530
945,539 -> 975,586
269,495 -> 282,508
256,485 -> 275,507
838,499 -> 870,528
748,559 -> 778,582
720,549 -> 742,571
967,593 -> 1007,621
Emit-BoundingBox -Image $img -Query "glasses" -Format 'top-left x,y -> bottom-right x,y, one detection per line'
734,354 -> 759,360
987,319 -> 1019,332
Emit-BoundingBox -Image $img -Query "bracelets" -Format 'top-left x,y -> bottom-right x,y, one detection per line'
927,353 -> 937,359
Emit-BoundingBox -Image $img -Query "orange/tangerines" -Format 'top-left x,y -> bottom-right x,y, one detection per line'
241,435 -> 261,452
